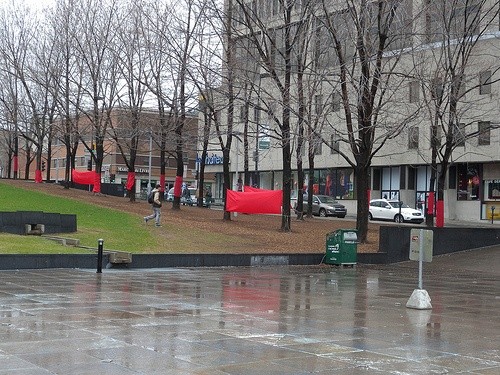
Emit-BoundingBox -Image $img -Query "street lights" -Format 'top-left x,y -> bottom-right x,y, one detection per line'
255,72 -> 272,189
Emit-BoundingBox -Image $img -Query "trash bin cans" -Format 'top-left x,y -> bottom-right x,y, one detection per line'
325,228 -> 357,268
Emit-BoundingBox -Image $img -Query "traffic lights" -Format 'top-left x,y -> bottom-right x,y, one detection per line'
42,162 -> 45,171
110,174 -> 115,182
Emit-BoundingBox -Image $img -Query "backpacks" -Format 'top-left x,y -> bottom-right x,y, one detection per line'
148,191 -> 154,204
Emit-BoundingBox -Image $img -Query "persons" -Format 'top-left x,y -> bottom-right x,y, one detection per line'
143,183 -> 164,227
183,182 -> 191,206
205,189 -> 212,209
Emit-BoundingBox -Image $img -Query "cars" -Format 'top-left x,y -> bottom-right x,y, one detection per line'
141,185 -> 211,207
368,198 -> 425,224
294,194 -> 347,218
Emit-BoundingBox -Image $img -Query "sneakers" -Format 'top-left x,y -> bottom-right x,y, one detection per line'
155,222 -> 163,227
144,216 -> 148,224
